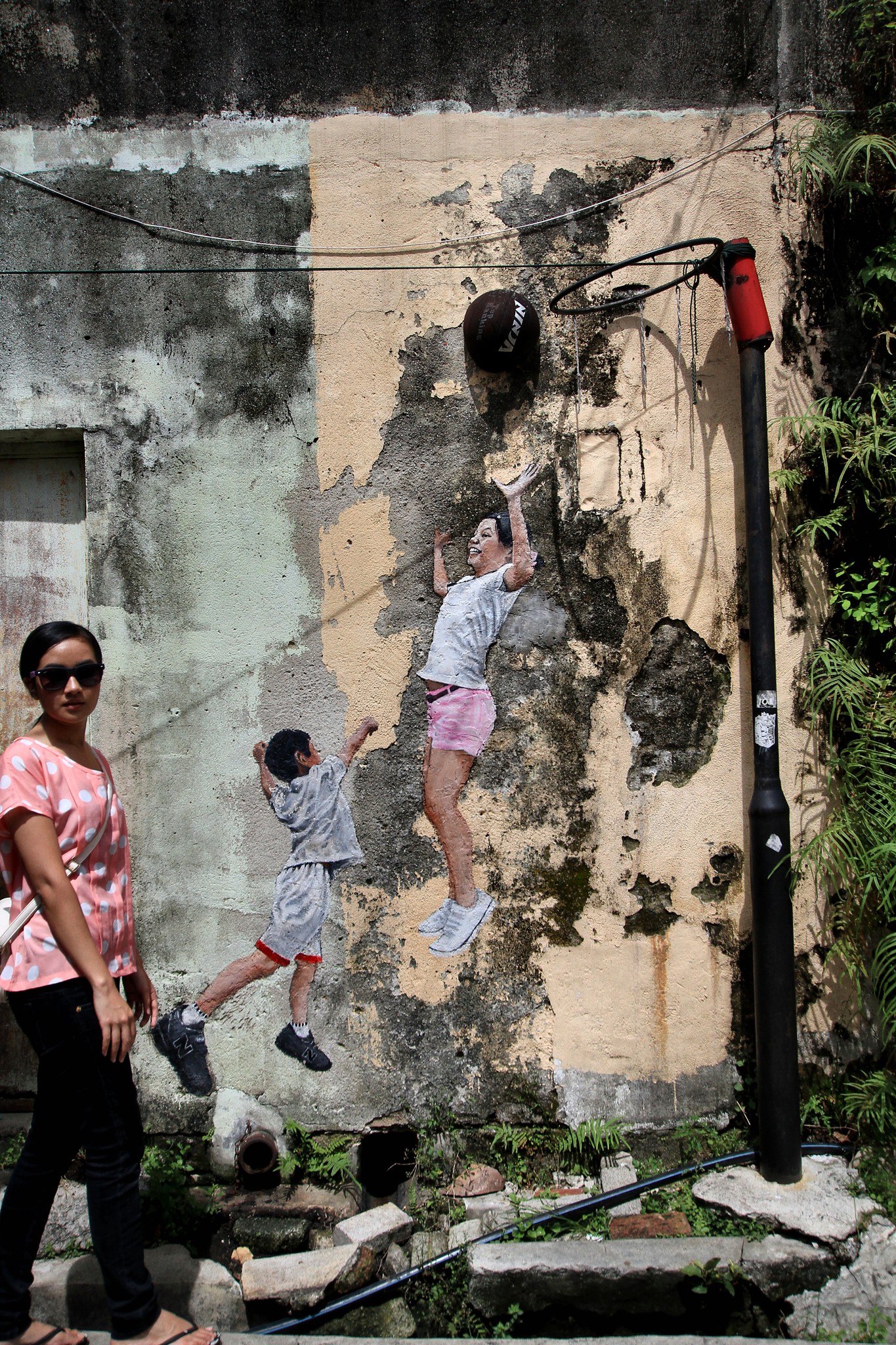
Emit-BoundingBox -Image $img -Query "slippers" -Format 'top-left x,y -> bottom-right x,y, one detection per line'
28,1323 -> 88,1345
161,1320 -> 220,1345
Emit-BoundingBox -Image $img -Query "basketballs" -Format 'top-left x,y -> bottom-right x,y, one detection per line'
462,288 -> 542,366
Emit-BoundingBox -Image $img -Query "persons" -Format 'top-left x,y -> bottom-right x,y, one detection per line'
0,620 -> 226,1345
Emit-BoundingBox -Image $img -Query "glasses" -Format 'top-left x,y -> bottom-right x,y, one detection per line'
29,663 -> 105,690
